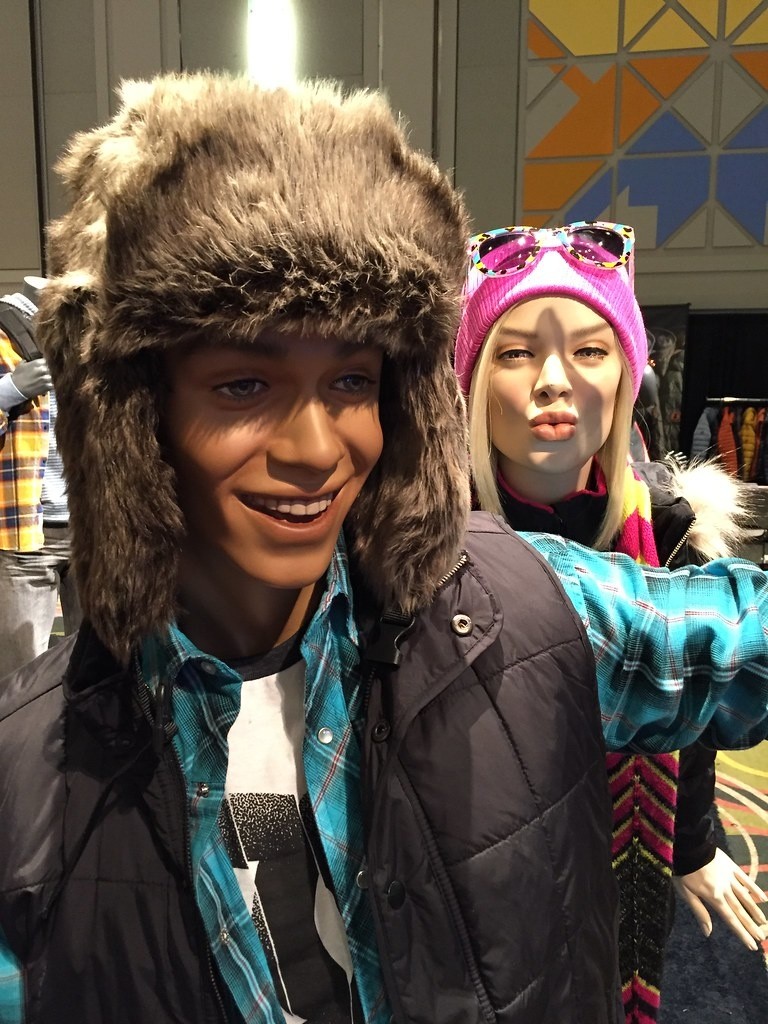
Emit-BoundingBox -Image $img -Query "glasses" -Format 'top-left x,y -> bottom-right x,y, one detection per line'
461,219 -> 635,305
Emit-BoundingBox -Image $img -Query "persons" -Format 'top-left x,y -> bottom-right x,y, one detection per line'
0,136 -> 768,1024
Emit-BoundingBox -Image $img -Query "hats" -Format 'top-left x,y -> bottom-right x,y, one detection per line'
36,73 -> 472,678
452,226 -> 645,402
